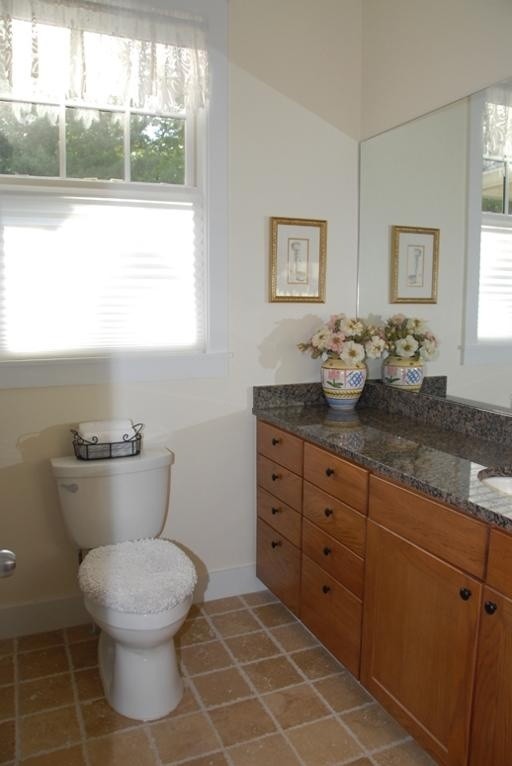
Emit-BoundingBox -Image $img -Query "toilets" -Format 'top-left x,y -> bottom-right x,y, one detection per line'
49,444 -> 199,721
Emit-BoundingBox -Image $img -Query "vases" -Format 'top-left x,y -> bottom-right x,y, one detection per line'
323,410 -> 366,453
320,358 -> 369,411
380,354 -> 424,395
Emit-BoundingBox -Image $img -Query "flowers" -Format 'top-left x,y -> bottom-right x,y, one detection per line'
384,315 -> 437,364
297,312 -> 384,366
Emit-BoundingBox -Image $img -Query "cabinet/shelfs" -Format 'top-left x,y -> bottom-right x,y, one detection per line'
256,418 -> 512,766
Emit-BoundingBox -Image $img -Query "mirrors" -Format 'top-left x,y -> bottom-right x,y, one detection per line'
358,77 -> 512,418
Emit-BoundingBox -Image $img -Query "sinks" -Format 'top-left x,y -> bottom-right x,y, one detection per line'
476,461 -> 512,499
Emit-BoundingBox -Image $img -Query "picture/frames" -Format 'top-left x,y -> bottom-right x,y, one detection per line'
391,225 -> 440,305
268,216 -> 328,302
